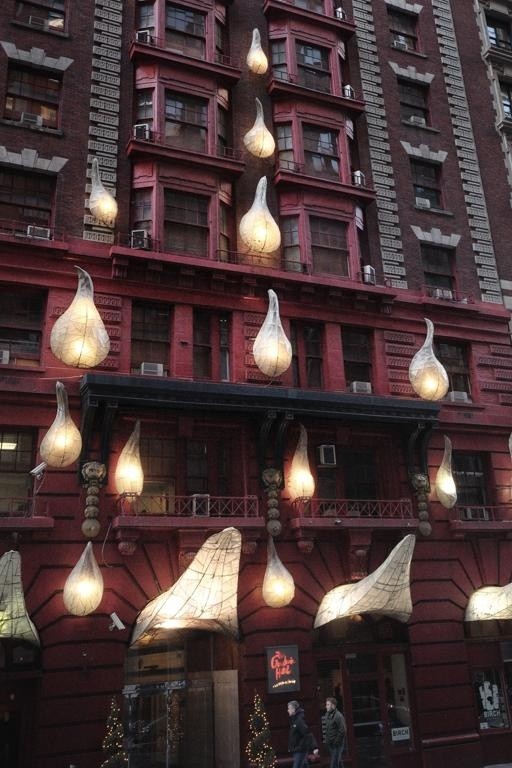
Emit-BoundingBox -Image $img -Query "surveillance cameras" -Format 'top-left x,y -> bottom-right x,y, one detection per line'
110,610 -> 127,631
29,462 -> 47,477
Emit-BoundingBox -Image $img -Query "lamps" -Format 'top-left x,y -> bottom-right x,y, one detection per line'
48,263 -> 113,369
464,582 -> 512,623
314,533 -> 417,630
113,418 -> 145,503
252,287 -> 314,610
39,379 -> 83,471
237,26 -> 283,254
130,526 -> 243,653
60,541 -> 104,618
89,158 -> 118,224
409,317 -> 459,510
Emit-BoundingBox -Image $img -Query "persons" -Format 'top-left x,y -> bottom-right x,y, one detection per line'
324,697 -> 348,768
286,700 -> 320,768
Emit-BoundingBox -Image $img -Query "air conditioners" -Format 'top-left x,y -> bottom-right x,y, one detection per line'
135,29 -> 151,44
363,265 -> 376,283
447,389 -> 468,403
140,361 -> 165,376
130,228 -> 150,249
345,83 -> 356,98
353,168 -> 366,186
131,123 -> 151,139
26,223 -> 51,240
315,441 -> 338,466
350,380 -> 372,395
19,111 -> 43,128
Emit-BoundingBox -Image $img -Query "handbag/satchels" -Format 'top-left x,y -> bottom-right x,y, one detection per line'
307,753 -> 321,764
